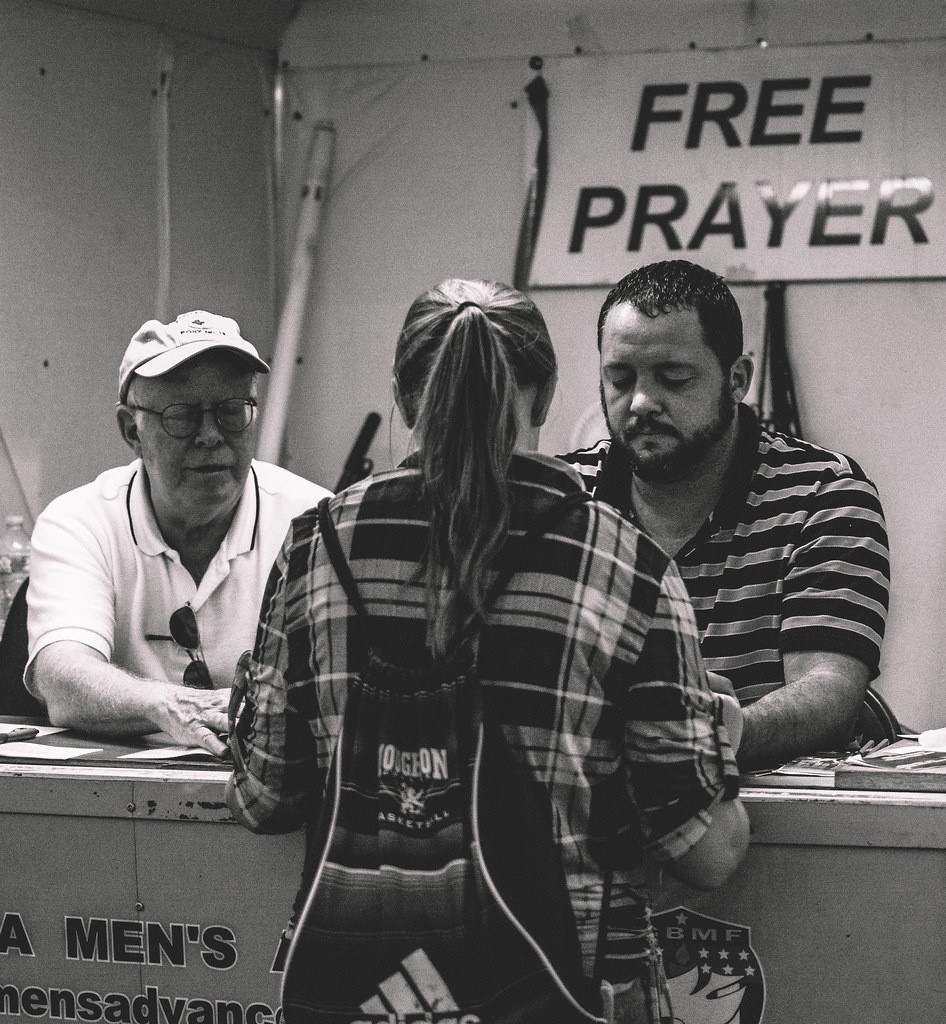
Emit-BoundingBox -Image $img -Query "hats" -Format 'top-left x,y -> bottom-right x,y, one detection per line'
117,310 -> 271,402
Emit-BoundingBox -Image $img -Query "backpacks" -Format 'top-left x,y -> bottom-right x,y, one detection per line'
280,492 -> 612,1024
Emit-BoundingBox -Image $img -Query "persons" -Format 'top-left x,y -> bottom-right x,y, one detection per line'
552,259 -> 891,772
22,309 -> 338,758
224,277 -> 752,1024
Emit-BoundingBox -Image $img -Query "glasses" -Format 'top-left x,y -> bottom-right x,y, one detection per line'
145,600 -> 214,691
126,393 -> 257,439
